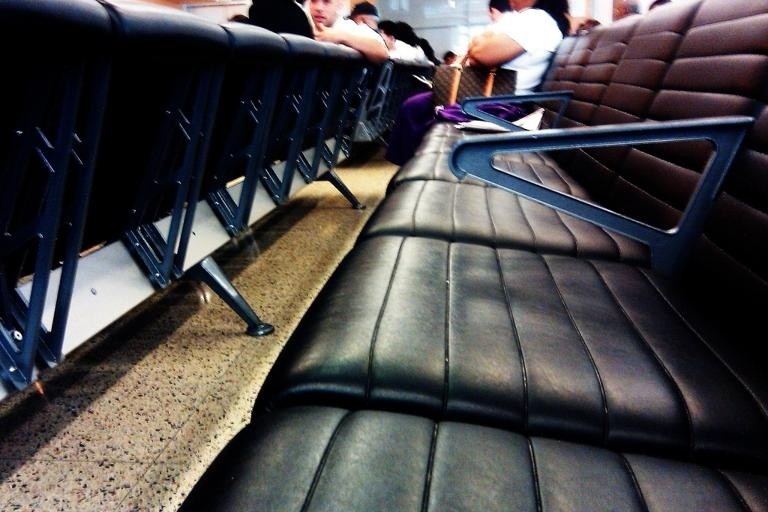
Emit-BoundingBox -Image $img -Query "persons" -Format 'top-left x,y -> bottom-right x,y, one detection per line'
388,0 -> 570,168
227,0 -> 442,66
613,1 -> 641,21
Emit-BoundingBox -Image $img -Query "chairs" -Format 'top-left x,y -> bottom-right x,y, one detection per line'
0,0 -> 767,512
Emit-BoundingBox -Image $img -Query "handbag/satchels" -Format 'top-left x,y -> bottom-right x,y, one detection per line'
432,56 -> 516,107
